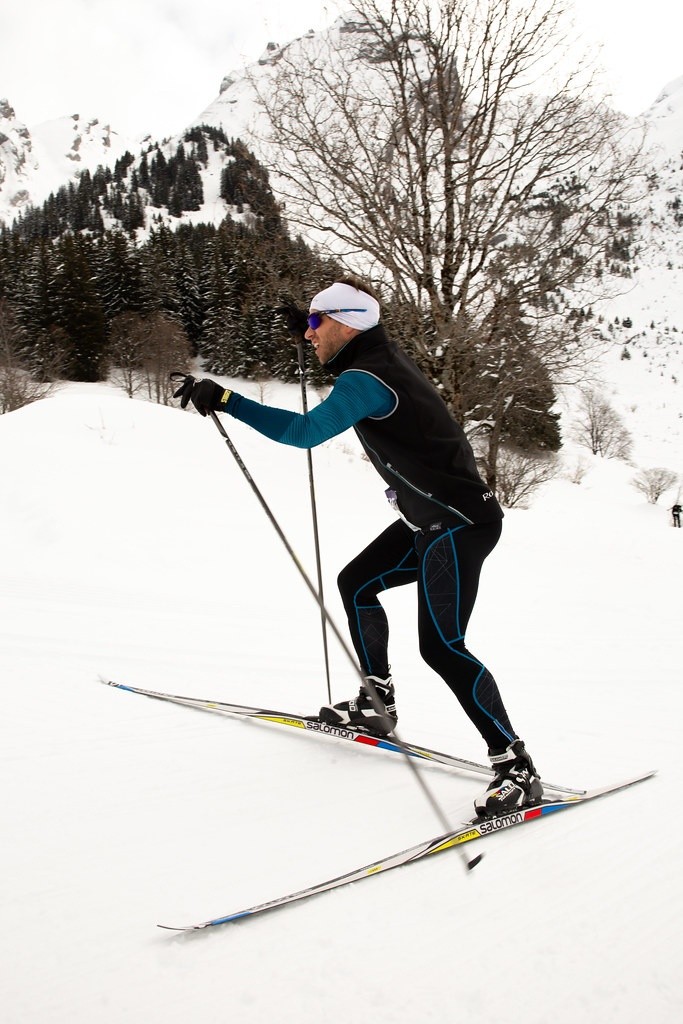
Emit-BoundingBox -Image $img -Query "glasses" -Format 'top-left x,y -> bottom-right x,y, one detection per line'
306,308 -> 367,330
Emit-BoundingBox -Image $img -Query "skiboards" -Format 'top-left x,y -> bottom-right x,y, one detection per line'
95,670 -> 662,932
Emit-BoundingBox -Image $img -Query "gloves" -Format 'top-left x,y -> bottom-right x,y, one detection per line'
173,375 -> 234,417
276,303 -> 309,337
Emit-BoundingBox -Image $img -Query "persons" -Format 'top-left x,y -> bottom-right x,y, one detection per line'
672,505 -> 683,529
174,277 -> 543,818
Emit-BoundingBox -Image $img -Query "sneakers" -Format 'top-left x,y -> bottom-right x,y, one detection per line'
319,673 -> 398,736
473,735 -> 544,817
